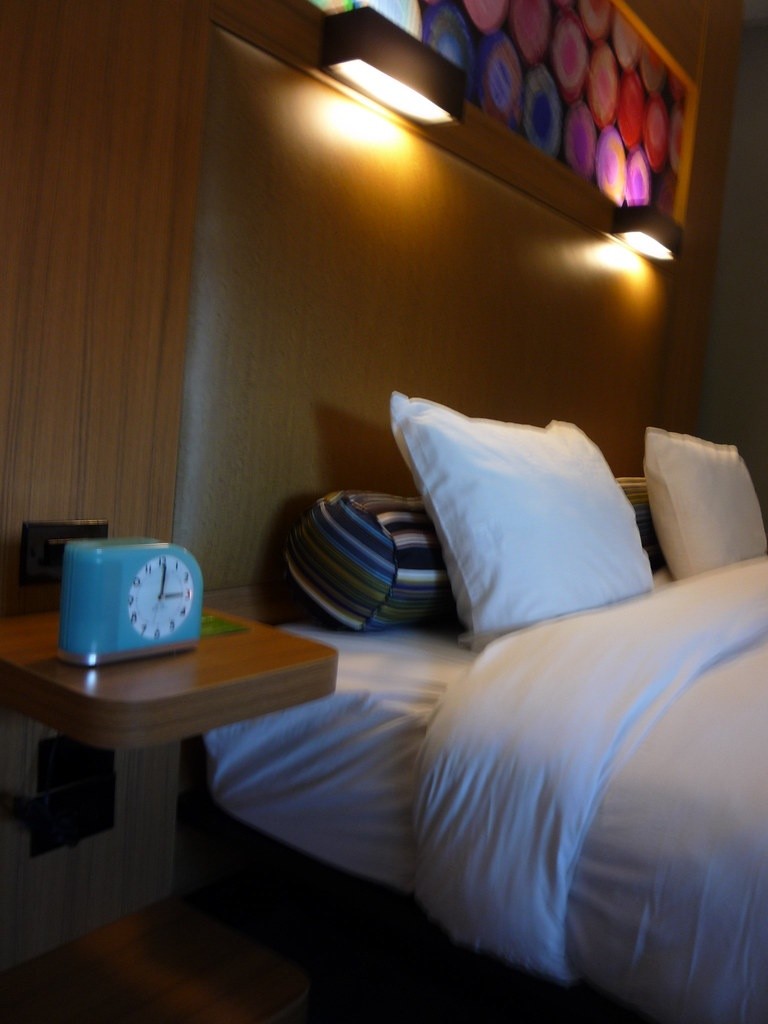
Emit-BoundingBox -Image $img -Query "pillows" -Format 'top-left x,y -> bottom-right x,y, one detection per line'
388,400 -> 651,648
286,490 -> 453,633
616,474 -> 667,575
644,424 -> 767,579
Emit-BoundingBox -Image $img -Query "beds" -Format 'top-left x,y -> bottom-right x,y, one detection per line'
202,551 -> 768,1024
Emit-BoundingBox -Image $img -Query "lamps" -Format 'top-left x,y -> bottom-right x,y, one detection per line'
611,203 -> 681,260
325,7 -> 464,127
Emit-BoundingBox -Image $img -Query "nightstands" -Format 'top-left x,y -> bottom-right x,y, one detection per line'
0,605 -> 337,1022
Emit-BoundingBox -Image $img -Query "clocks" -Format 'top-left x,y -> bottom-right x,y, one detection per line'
58,540 -> 204,669
